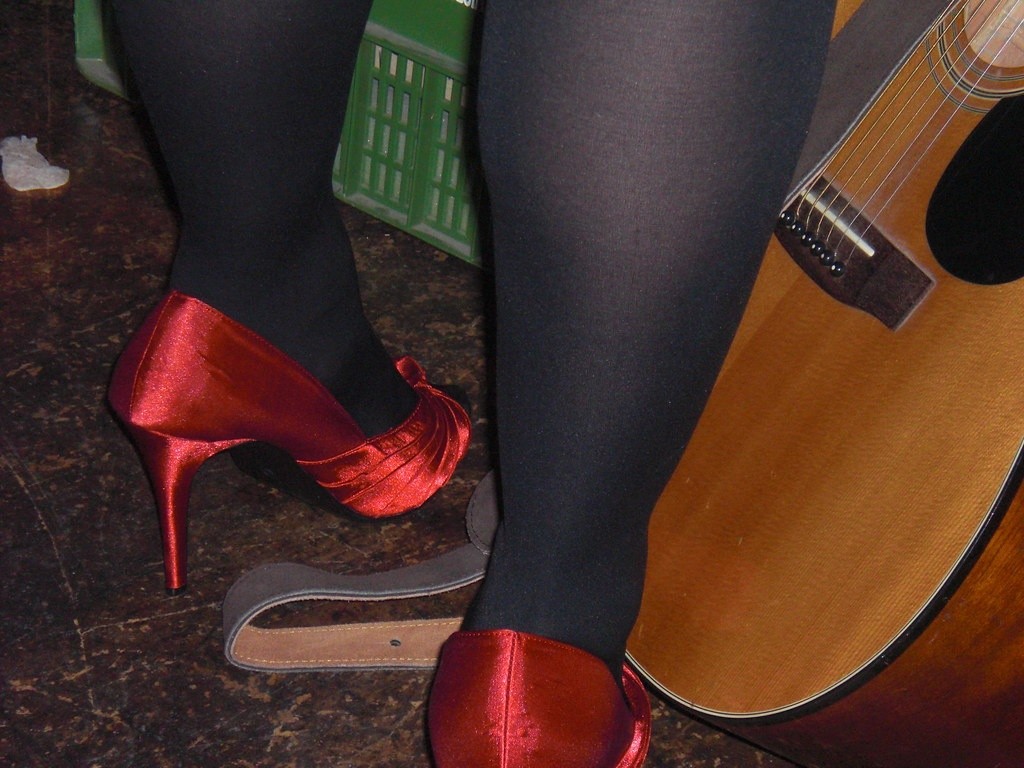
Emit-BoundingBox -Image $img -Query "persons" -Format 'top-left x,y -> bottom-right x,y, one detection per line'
97,0 -> 837,768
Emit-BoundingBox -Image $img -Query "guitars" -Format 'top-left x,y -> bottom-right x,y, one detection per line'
621,3 -> 1023,767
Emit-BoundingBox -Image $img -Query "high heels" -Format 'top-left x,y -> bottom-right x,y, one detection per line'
427,630 -> 653,768
105,289 -> 474,596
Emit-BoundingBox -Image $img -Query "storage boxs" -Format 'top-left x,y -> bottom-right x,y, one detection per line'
73,1 -> 494,275
